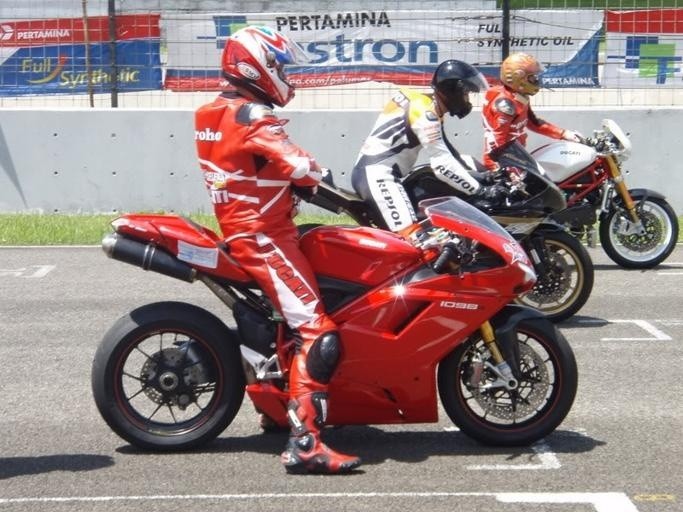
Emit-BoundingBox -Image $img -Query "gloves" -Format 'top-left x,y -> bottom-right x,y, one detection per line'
562,129 -> 587,145
481,183 -> 511,205
486,167 -> 506,181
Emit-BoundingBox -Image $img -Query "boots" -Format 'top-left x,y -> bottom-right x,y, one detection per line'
282,390 -> 362,473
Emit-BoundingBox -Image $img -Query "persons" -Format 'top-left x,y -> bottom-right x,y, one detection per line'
195,27 -> 366,476
483,53 -> 600,185
351,60 -> 510,232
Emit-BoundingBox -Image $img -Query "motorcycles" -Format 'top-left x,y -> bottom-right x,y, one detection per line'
92,194 -> 578,455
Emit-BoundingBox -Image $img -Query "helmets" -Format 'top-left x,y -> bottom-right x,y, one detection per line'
221,25 -> 312,108
500,52 -> 547,96
429,59 -> 491,118
